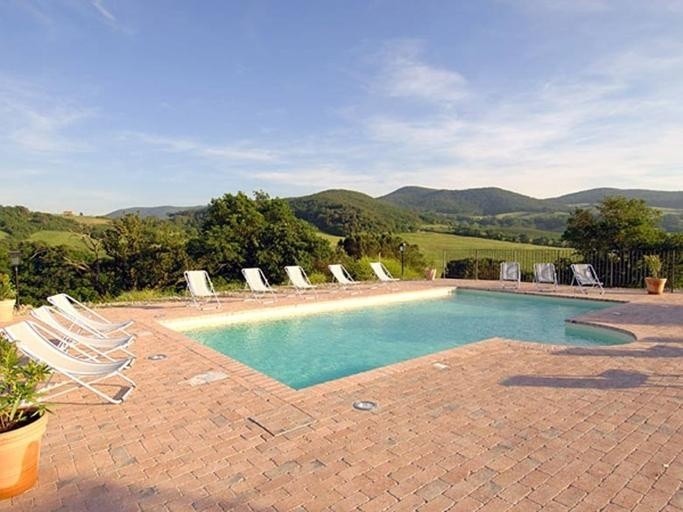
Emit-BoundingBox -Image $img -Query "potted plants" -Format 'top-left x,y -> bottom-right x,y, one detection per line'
637,251 -> 668,297
0,333 -> 56,501
420,252 -> 443,282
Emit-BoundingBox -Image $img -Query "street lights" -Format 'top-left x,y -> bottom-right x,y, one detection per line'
7,249 -> 21,310
397,242 -> 406,280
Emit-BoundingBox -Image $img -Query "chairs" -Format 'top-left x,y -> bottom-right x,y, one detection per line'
0,285 -> 137,409
369,260 -> 403,294
570,261 -> 606,296
532,262 -> 559,293
281,263 -> 320,302
325,262 -> 365,296
498,261 -> 521,293
180,267 -> 223,313
239,266 -> 281,306
